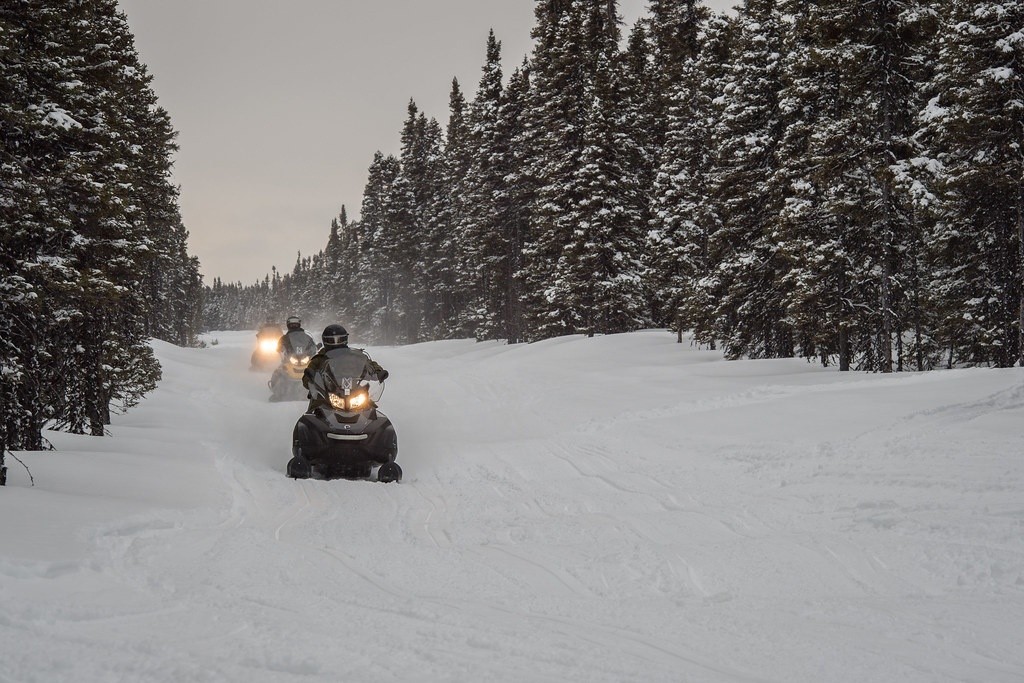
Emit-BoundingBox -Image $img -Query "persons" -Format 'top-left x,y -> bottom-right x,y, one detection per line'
269,316 -> 318,390
301,324 -> 389,416
255,318 -> 284,362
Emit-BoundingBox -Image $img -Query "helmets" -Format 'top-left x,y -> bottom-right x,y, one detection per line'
266,316 -> 275,325
322,324 -> 349,348
287,317 -> 301,329
